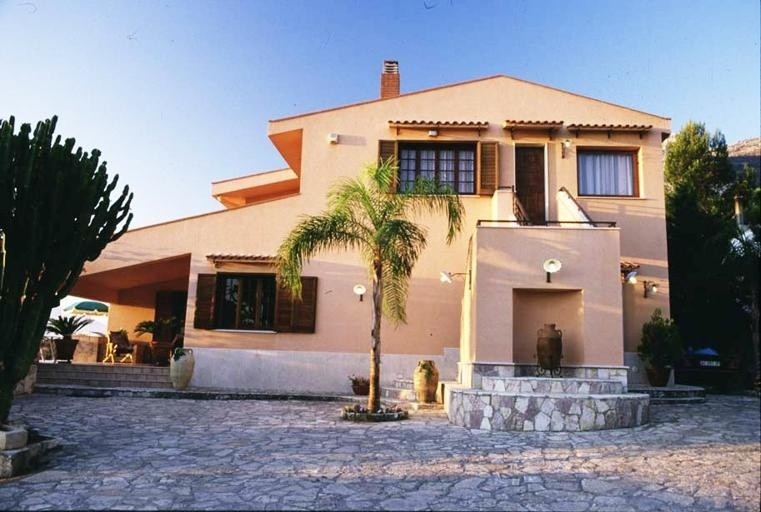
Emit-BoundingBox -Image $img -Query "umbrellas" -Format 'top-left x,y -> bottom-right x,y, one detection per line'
64,301 -> 108,332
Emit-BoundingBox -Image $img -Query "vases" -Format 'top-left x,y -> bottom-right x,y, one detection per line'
535,321 -> 567,378
413,358 -> 440,403
169,345 -> 196,390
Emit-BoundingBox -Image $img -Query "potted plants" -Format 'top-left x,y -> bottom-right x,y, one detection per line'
635,308 -> 684,387
47,314 -> 96,363
349,373 -> 373,395
134,315 -> 180,365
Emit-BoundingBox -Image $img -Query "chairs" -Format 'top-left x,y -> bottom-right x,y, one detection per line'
102,328 -> 135,365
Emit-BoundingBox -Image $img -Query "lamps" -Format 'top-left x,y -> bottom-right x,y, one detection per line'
643,279 -> 660,298
543,257 -> 563,284
437,267 -> 472,288
560,139 -> 571,158
618,267 -> 638,287
353,283 -> 368,302
